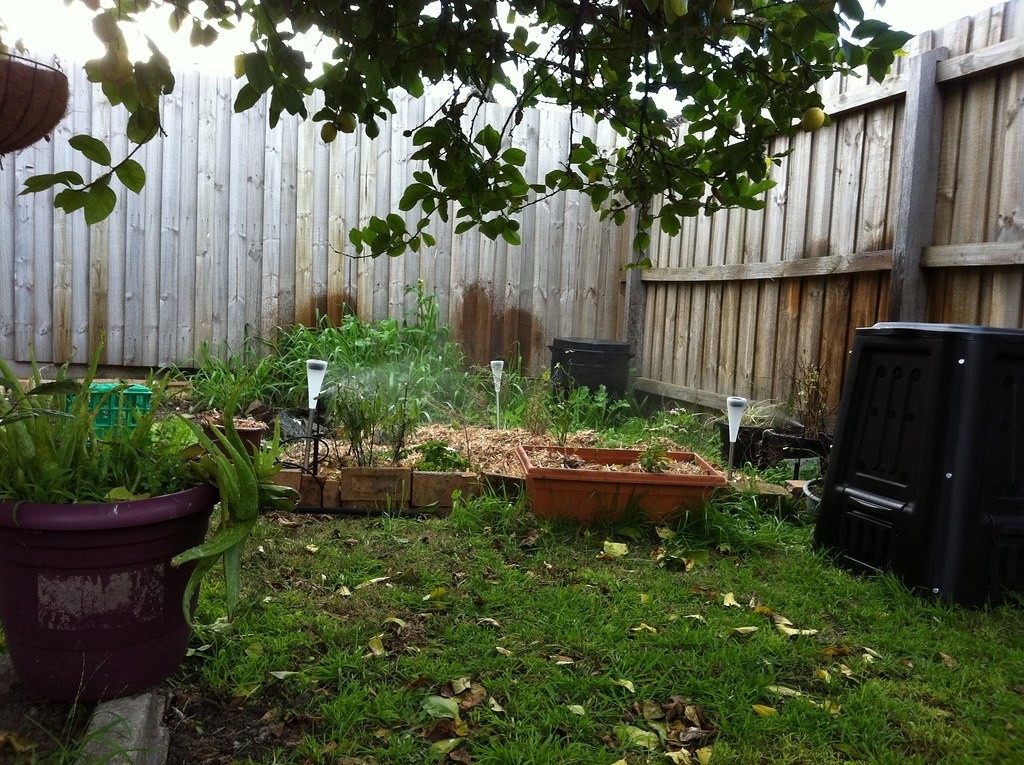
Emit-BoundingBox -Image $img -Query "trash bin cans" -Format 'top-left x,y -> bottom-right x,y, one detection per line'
551,337 -> 633,420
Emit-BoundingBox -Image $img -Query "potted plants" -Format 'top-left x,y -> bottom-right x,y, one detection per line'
1,338 -> 219,703
162,339 -> 292,465
256,375 -> 728,524
691,396 -> 793,472
784,429 -> 833,516
763,348 -> 834,472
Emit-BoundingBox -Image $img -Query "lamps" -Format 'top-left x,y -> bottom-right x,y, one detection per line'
306,359 -> 327,409
490,361 -> 504,393
726,397 -> 747,442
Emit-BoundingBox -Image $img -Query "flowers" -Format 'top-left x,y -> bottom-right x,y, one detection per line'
635,408 -> 688,472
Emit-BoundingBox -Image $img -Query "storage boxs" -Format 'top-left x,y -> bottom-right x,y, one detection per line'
65,380 -> 153,438
